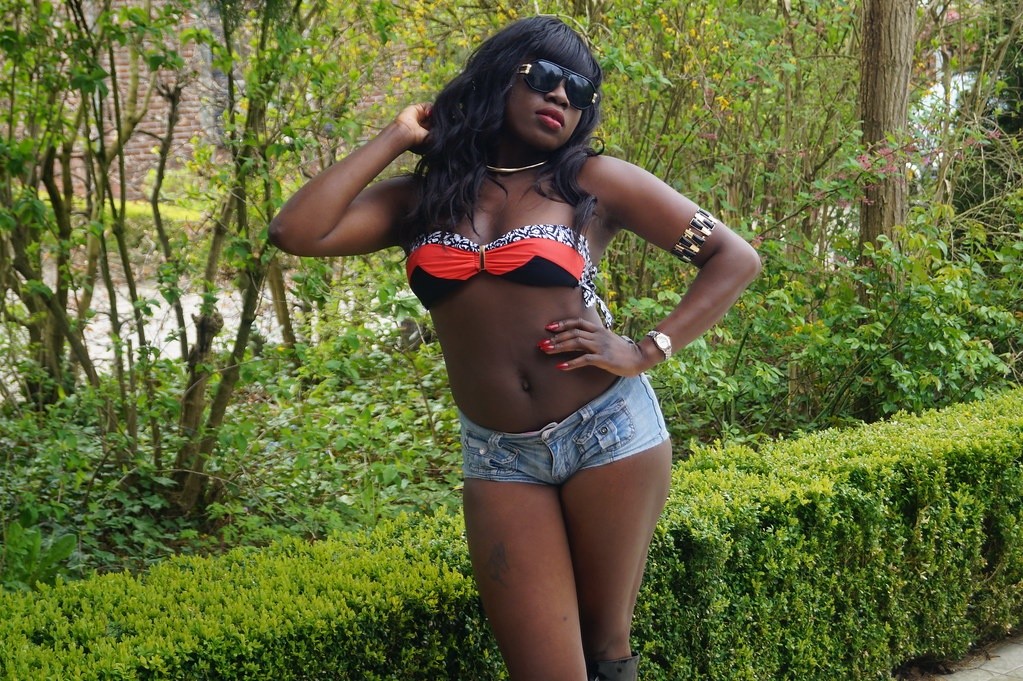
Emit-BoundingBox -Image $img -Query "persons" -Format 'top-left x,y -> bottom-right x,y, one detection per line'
269,16 -> 762,681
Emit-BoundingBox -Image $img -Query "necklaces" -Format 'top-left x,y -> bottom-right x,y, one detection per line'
486,158 -> 547,172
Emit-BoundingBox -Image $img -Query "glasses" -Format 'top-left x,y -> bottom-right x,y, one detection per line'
515,58 -> 599,110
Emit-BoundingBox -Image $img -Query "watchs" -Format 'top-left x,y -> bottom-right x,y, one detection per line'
647,331 -> 672,360
